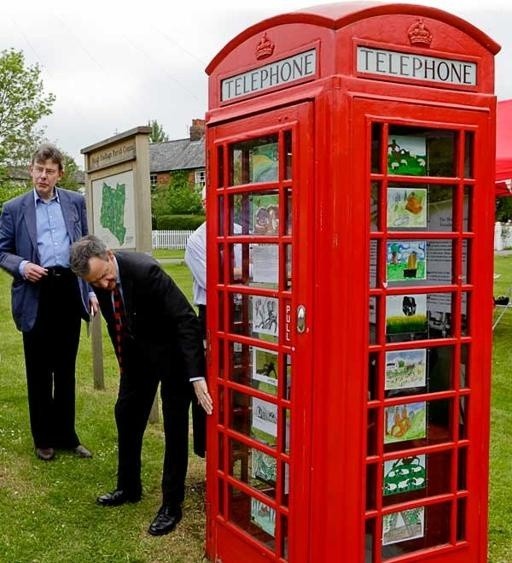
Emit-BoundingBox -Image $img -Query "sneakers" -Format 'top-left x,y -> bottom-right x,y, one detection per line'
37,447 -> 55,461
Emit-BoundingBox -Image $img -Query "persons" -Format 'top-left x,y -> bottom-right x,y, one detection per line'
68,233 -> 216,536
183,180 -> 251,458
0,143 -> 103,464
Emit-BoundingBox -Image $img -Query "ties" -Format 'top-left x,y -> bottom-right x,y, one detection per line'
112,283 -> 126,366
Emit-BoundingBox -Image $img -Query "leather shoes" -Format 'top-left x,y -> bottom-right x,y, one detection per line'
73,444 -> 93,457
147,506 -> 183,536
95,489 -> 141,505
